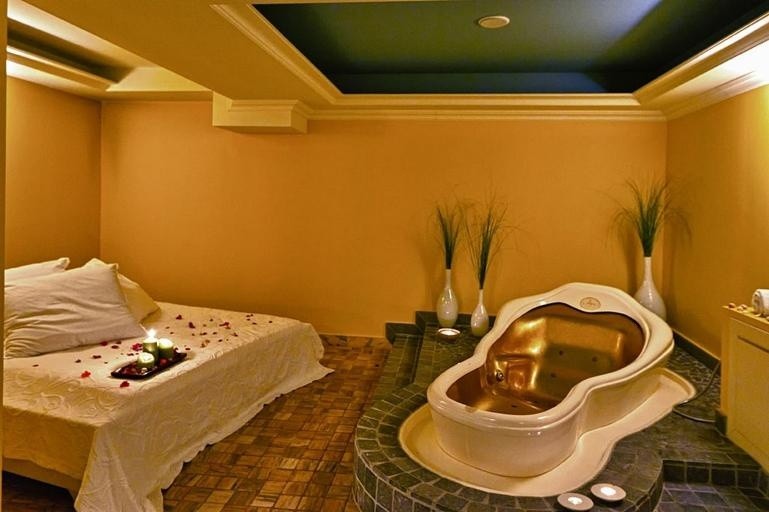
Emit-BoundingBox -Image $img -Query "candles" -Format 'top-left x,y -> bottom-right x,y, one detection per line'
136,351 -> 155,369
158,340 -> 172,359
142,338 -> 159,363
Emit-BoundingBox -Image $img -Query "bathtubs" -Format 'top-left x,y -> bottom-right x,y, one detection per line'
427,279 -> 675,475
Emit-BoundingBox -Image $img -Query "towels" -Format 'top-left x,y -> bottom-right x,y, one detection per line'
752,287 -> 769,314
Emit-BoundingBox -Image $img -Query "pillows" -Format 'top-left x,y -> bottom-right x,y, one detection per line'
4,263 -> 152,359
81,258 -> 160,324
4,255 -> 69,282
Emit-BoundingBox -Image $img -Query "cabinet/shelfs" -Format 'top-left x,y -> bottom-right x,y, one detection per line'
722,301 -> 769,480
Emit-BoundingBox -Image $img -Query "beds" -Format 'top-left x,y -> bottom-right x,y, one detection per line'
4,257 -> 335,511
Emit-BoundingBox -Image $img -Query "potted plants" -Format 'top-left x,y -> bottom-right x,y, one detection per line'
607,177 -> 695,325
457,194 -> 521,339
425,200 -> 465,331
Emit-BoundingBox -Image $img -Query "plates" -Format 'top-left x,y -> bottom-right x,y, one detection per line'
109,352 -> 187,380
